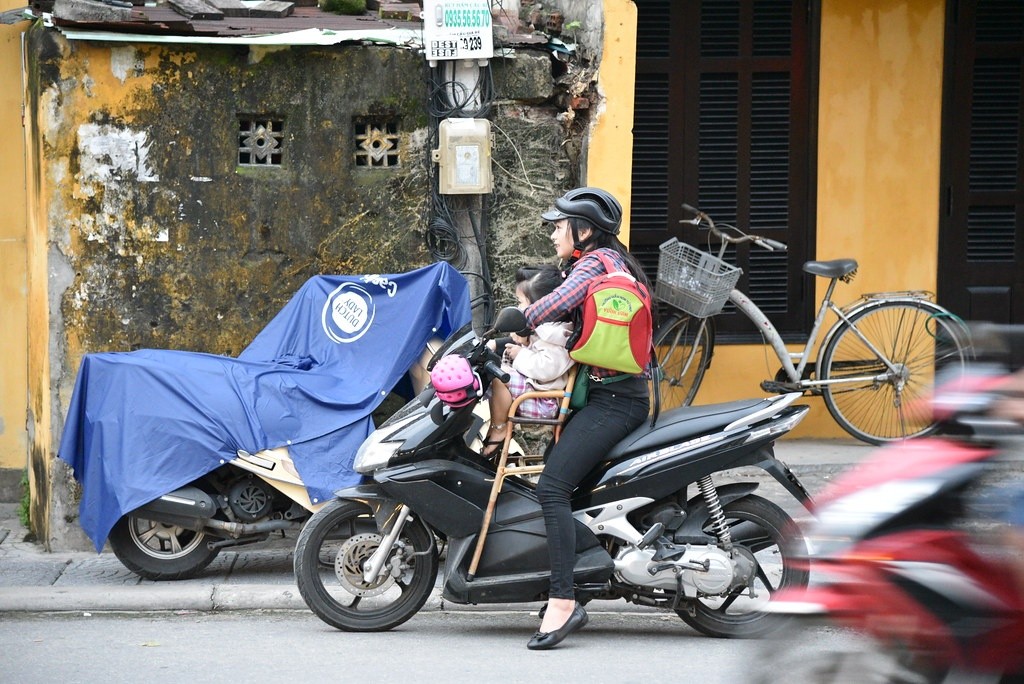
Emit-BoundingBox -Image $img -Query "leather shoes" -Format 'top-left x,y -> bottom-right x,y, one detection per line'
526,601 -> 588,650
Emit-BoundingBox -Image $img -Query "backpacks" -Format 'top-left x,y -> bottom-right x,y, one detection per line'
568,252 -> 658,377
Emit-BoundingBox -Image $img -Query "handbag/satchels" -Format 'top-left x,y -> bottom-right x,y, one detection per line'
568,363 -> 594,411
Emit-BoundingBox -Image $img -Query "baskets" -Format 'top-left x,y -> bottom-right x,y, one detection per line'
655,236 -> 744,318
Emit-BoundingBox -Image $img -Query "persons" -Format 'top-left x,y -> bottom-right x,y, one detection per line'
473,187 -> 650,651
482,263 -> 574,457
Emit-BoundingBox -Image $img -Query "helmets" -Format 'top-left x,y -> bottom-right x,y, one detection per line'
541,187 -> 623,235
430,352 -> 482,408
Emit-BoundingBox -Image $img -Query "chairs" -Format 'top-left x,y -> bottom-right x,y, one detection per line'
469,369 -> 576,580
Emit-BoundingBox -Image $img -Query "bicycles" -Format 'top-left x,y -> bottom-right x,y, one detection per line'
649,202 -> 976,446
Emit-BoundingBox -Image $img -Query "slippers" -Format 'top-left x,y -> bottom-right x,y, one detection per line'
482,431 -> 517,460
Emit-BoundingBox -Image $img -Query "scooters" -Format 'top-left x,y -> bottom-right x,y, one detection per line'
780,375 -> 1023,683
83,260 -> 447,580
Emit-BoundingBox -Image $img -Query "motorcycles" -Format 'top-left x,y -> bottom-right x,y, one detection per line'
292,306 -> 816,641
